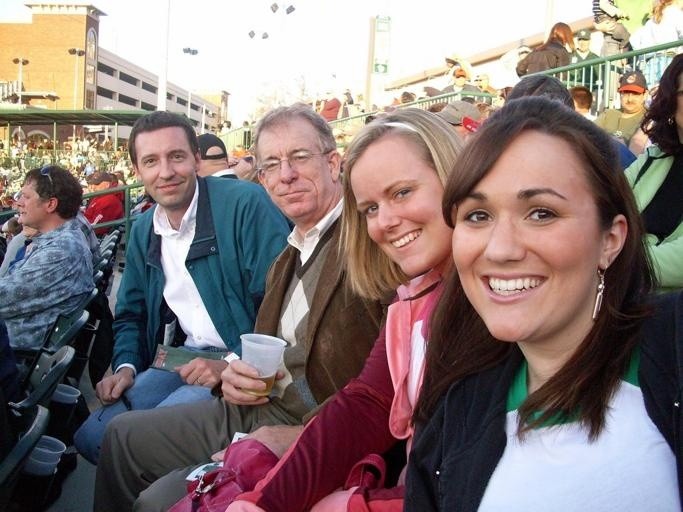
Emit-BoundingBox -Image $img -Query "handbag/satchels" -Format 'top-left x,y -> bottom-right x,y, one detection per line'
165,436 -> 279,512
308,450 -> 406,512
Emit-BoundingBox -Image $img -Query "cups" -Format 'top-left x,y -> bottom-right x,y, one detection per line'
238,332 -> 288,399
50,383 -> 81,403
23,434 -> 67,464
22,455 -> 61,476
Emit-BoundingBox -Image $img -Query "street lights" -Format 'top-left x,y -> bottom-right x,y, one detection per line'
11,58 -> 31,151
182,47 -> 196,118
68,46 -> 84,156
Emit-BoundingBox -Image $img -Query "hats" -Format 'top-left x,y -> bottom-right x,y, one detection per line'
452,68 -> 466,79
192,133 -> 227,160
576,29 -> 592,41
88,172 -> 112,185
616,70 -> 647,95
432,99 -> 482,126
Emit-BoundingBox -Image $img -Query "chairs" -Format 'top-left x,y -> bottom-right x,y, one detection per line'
0,224 -> 128,511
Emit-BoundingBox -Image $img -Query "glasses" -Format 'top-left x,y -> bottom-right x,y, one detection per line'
253,147 -> 332,174
39,165 -> 55,199
